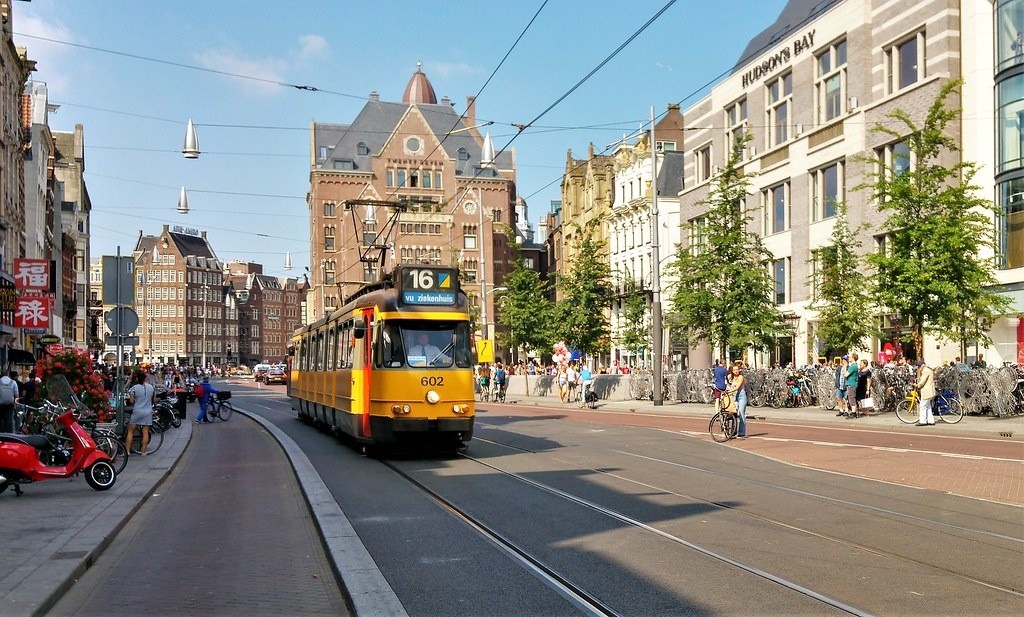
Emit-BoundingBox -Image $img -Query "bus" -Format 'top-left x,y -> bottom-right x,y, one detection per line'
286,265 -> 476,458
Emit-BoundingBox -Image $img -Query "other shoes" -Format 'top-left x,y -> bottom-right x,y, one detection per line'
835,410 -> 869,420
730,435 -> 746,439
561,398 -> 587,404
195,420 -> 207,424
915,421 -> 935,426
119,451 -> 147,456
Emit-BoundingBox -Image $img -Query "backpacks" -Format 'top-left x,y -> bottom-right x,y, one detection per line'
0,379 -> 15,404
195,383 -> 205,398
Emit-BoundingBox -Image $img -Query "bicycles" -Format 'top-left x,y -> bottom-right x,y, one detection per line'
575,382 -> 597,408
0,360 -> 233,496
710,388 -> 737,442
489,382 -> 508,402
634,362 -> 1024,426
477,379 -> 490,400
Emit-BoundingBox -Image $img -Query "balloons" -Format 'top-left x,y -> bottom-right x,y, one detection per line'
551,342 -> 573,372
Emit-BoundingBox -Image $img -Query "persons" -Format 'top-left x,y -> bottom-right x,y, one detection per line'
118,371 -> 157,458
855,360 -> 874,418
11,360 -> 289,407
844,353 -> 861,420
407,330 -> 453,368
914,360 -> 938,427
724,363 -> 749,439
714,360 -> 730,419
382,319 -> 403,366
0,367 -> 20,434
714,353 -> 989,375
194,376 -> 222,424
836,356 -> 852,416
472,353 -> 666,411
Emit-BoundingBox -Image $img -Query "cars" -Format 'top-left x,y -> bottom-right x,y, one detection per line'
252,364 -> 287,385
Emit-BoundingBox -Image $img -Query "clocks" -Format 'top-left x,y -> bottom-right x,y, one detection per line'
462,200 -> 477,215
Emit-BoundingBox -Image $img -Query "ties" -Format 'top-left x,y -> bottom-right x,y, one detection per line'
422,347 -> 427,361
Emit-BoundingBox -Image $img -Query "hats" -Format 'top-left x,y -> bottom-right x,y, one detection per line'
841,355 -> 850,360
916,361 -> 926,366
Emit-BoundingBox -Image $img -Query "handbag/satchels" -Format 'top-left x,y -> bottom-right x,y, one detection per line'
861,392 -> 874,409
